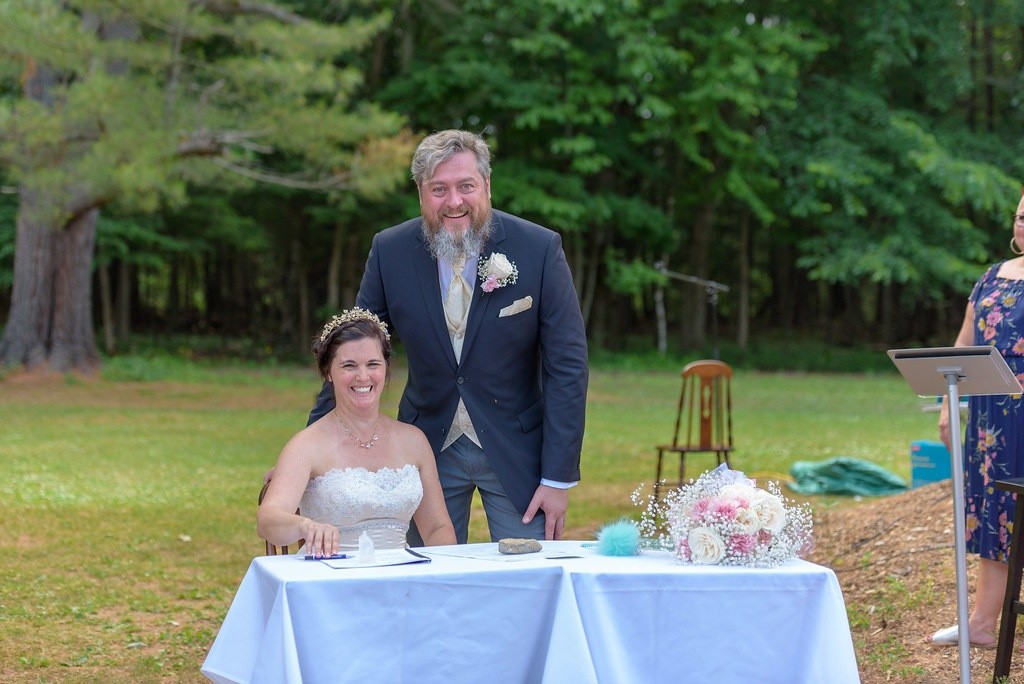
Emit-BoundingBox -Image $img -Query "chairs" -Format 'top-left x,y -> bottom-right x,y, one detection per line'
653,359 -> 736,523
257,479 -> 305,555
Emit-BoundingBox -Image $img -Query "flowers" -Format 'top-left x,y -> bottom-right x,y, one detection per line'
628,462 -> 818,568
477,251 -> 519,297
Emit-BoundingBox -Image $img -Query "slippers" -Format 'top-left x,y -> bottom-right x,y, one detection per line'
924,625 -> 999,649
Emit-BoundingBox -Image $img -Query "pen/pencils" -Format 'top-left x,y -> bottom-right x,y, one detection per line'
294,553 -> 356,561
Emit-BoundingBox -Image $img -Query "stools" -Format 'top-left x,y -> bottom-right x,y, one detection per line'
993,476 -> 1024,684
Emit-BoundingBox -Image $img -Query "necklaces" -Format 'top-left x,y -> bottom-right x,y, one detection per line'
330,406 -> 386,449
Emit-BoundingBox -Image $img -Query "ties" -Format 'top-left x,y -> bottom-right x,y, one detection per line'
444,253 -> 474,330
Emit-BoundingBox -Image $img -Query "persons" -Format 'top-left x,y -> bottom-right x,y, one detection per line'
253,305 -> 458,559
307,130 -> 591,549
926,182 -> 1024,648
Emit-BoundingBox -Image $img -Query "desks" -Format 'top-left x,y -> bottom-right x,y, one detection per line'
198,541 -> 861,683
